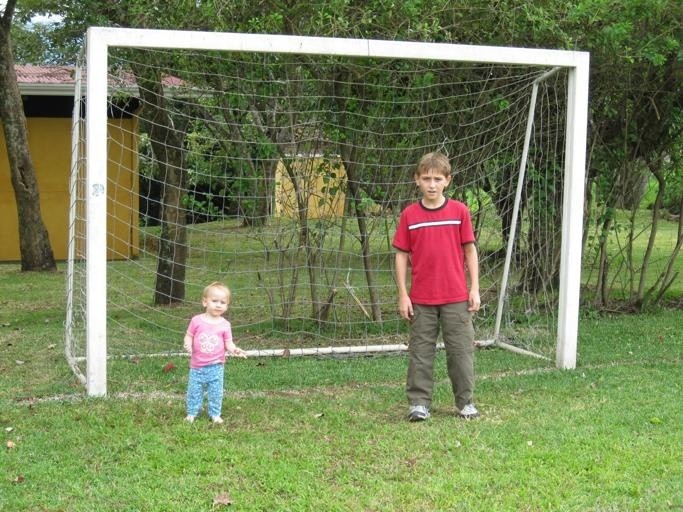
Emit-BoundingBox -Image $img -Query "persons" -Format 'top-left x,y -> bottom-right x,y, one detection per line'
182,281 -> 249,424
389,152 -> 482,420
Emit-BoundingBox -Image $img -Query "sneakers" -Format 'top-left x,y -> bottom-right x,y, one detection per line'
459,402 -> 478,415
408,405 -> 430,420
